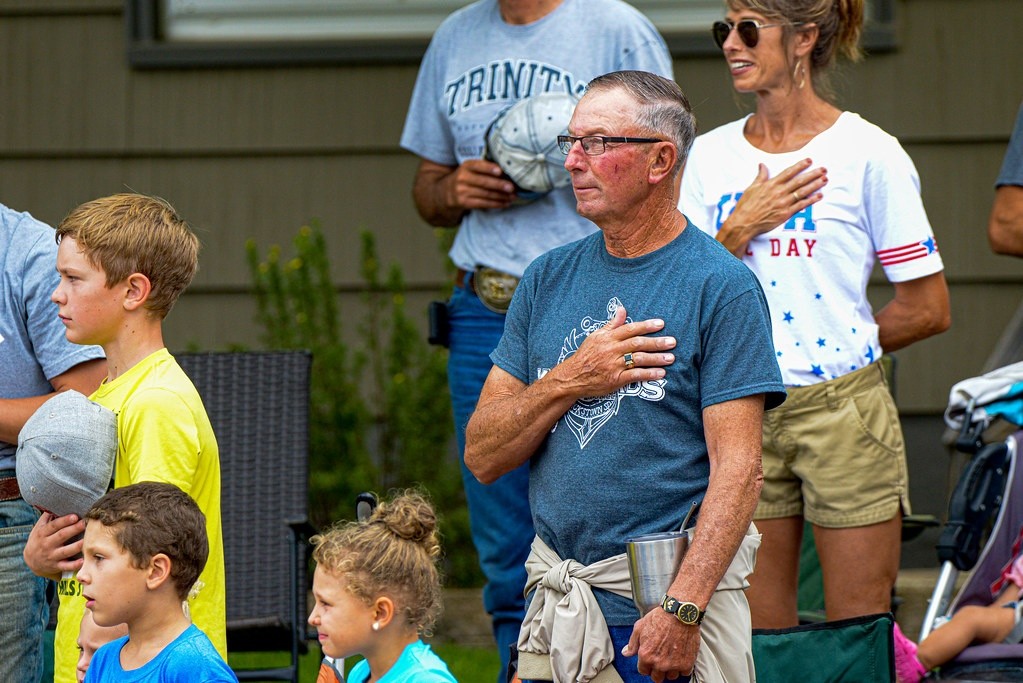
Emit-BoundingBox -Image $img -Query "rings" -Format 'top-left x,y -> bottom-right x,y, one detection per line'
793,192 -> 800,201
623,352 -> 635,369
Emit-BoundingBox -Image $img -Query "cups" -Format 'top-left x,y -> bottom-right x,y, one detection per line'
624,530 -> 689,618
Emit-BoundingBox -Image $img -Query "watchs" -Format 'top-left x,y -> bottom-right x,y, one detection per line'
660,593 -> 706,626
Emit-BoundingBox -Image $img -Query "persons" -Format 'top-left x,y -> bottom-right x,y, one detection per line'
463,70 -> 786,683
0,197 -> 106,683
396,0 -> 679,683
677,0 -> 954,629
22,194 -> 226,683
75,607 -> 128,683
306,487 -> 462,683
893,552 -> 1023,683
988,95 -> 1023,257
76,480 -> 238,683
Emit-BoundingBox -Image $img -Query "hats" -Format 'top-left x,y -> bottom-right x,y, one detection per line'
481,93 -> 580,204
16,389 -> 118,560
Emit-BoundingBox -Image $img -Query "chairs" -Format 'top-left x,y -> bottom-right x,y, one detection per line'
170,351 -> 318,683
752,355 -> 938,683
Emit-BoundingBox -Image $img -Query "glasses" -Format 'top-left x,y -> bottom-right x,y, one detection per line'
709,20 -> 805,49
557,134 -> 660,155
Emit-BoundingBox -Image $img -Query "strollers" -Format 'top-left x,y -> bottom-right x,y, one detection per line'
906,430 -> 1023,683
